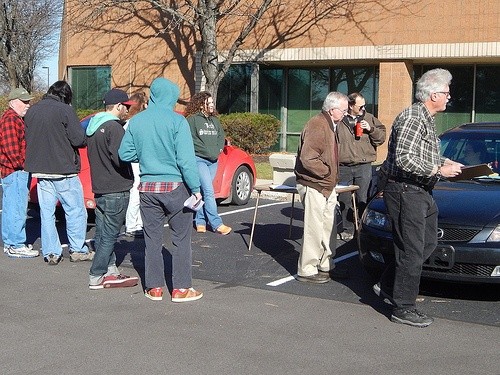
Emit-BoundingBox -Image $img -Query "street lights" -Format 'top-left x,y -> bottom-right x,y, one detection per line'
42,66 -> 50,89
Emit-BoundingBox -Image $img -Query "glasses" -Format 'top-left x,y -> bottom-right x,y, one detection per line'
436,91 -> 450,96
357,104 -> 366,109
338,108 -> 347,115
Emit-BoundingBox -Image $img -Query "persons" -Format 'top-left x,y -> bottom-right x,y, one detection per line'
294,92 -> 349,284
85,88 -> 139,289
333,93 -> 386,239
118,77 -> 204,302
462,142 -> 489,166
0,87 -> 40,258
184,91 -> 232,235
373,68 -> 465,327
24,80 -> 95,265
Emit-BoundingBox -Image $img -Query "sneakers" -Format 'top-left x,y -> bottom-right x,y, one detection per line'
373,282 -> 394,305
391,308 -> 434,328
144,287 -> 163,301
172,287 -> 204,302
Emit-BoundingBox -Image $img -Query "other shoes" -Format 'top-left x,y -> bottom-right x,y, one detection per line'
215,224 -> 232,235
196,226 -> 206,233
70,250 -> 95,262
47,254 -> 59,265
89,276 -> 104,289
296,271 -> 331,284
337,231 -> 354,239
125,230 -> 143,238
102,273 -> 139,287
3,246 -> 39,258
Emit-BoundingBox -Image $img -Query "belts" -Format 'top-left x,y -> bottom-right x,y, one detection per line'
388,175 -> 434,190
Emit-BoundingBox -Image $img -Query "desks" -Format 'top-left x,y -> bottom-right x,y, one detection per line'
248,184 -> 363,260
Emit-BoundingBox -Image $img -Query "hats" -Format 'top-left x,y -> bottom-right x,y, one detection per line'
102,89 -> 138,105
8,88 -> 36,102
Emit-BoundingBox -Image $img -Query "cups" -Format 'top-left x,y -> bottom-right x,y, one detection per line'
356,121 -> 363,137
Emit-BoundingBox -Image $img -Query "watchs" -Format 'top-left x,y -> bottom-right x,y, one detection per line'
436,166 -> 442,178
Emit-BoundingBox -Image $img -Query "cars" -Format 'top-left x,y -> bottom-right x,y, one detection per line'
28,112 -> 257,212
355,121 -> 500,289
